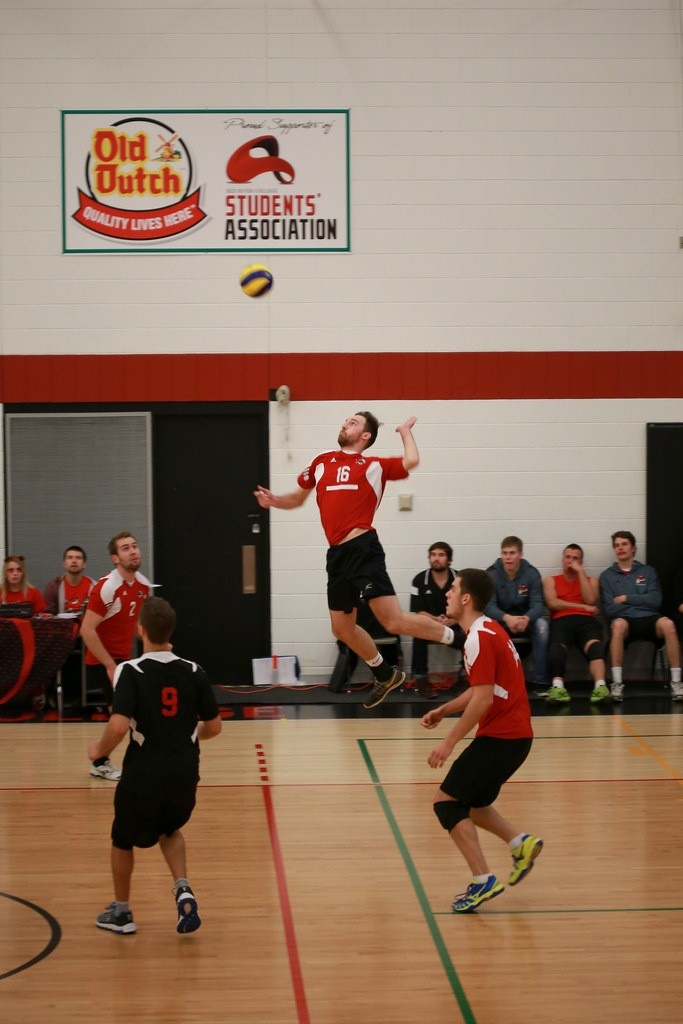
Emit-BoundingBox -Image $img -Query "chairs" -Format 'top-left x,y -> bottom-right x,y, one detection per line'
328,631 -> 398,694
80,639 -> 114,710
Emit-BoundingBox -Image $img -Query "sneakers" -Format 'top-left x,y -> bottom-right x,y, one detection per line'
589,684 -> 613,704
506,834 -> 544,886
670,680 -> 683,700
89,757 -> 125,780
94,901 -> 139,934
547,686 -> 571,704
451,875 -> 505,912
363,664 -> 407,709
175,885 -> 202,934
610,682 -> 626,701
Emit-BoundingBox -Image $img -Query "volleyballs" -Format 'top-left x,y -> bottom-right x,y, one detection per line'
240,264 -> 273,297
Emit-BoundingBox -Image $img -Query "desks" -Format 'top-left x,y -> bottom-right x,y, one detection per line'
0,617 -> 83,718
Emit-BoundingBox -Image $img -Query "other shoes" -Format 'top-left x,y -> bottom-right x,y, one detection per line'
32,683 -> 47,712
417,680 -> 439,698
533,683 -> 551,697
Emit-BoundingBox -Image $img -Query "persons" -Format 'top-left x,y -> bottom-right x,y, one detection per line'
0,555 -> 47,614
404,542 -> 462,691
80,532 -> 164,781
419,568 -> 543,912
43,545 -> 98,616
254,412 -> 467,709
482,536 -> 550,697
599,531 -> 683,702
87,596 -> 221,934
543,544 -> 610,702
